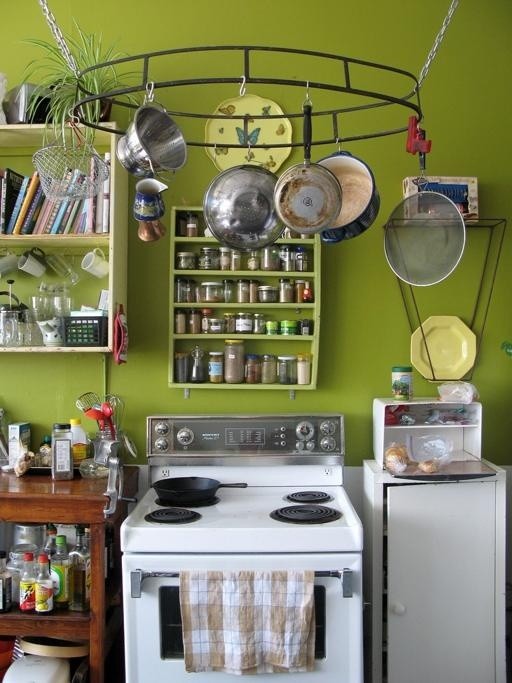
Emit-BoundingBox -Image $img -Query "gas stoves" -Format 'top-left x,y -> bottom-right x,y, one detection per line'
124,479 -> 362,532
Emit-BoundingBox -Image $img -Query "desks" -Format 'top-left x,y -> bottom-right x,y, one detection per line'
0,466 -> 141,683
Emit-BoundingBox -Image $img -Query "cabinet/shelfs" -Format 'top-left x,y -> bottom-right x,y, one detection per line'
0,120 -> 128,355
167,205 -> 321,391
363,395 -> 507,683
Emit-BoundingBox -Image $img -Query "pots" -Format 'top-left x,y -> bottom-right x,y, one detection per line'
203,99 -> 381,253
150,474 -> 249,503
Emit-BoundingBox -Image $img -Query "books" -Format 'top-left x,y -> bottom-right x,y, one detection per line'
0,151 -> 112,235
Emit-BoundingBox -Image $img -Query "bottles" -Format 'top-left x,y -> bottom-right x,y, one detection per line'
173,209 -> 314,386
41,417 -> 91,480
0,521 -> 116,616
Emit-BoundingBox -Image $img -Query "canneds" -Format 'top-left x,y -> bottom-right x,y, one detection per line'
174,211 -> 311,384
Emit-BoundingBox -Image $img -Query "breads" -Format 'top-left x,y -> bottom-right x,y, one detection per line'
384,442 -> 409,476
418,460 -> 439,473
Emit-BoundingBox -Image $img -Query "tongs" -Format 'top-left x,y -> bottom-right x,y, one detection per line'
100,456 -> 123,516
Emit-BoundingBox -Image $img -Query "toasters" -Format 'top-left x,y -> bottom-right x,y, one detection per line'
1,81 -> 54,126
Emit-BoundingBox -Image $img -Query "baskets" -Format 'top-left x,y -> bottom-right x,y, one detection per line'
63,315 -> 109,347
32,146 -> 109,204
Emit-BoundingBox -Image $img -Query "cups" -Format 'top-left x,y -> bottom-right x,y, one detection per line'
93,431 -> 121,465
0,243 -> 109,347
133,176 -> 170,223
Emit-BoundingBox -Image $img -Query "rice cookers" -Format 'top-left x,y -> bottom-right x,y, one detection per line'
3,655 -> 72,682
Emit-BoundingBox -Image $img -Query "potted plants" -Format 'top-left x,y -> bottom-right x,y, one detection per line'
13,19 -> 144,166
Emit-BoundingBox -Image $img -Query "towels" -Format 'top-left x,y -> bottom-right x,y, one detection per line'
177,570 -> 316,673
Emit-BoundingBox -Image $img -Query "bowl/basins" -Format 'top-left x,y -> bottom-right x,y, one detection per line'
80,458 -> 109,480
114,99 -> 190,185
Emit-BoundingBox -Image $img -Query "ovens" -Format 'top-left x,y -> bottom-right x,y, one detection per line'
121,553 -> 366,683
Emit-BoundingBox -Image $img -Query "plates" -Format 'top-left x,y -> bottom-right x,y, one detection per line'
204,92 -> 295,174
408,315 -> 478,384
22,465 -> 79,473
